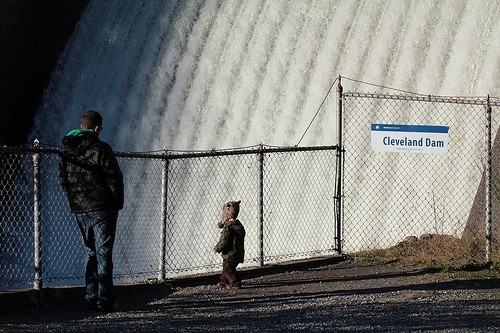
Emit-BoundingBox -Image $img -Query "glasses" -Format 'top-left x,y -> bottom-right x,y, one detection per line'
99,126 -> 103,130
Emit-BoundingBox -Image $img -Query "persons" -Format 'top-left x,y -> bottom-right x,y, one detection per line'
60,110 -> 125,311
213,200 -> 246,289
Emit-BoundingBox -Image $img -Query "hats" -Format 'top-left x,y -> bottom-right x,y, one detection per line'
218,201 -> 241,229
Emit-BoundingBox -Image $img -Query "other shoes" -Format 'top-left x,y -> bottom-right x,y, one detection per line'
226,285 -> 240,290
102,306 -> 120,314
